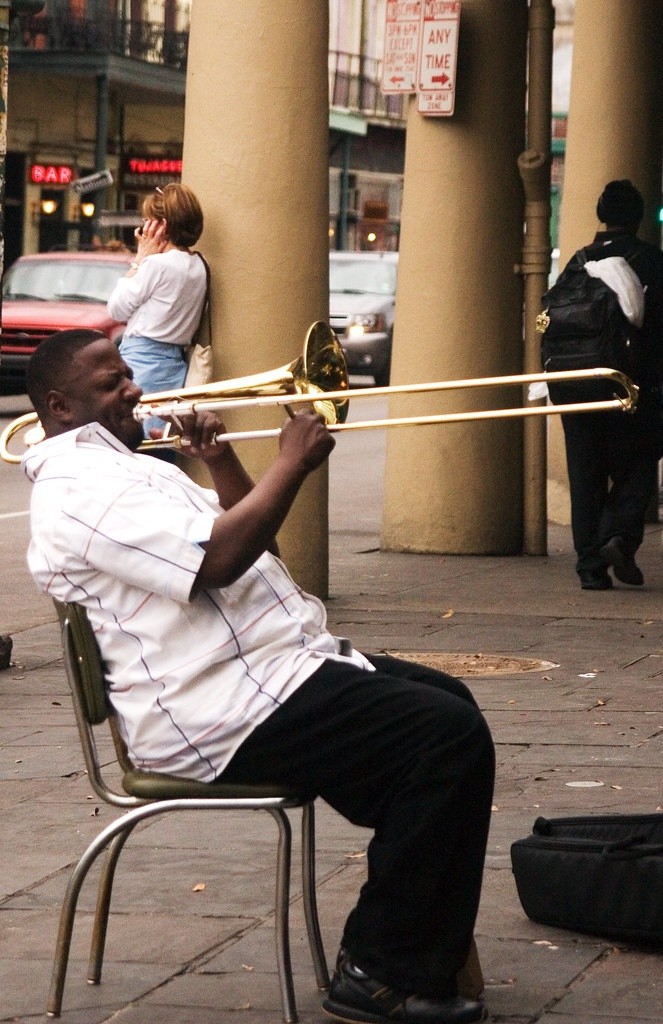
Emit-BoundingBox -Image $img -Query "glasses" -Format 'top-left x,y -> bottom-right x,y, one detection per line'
154,186 -> 163,196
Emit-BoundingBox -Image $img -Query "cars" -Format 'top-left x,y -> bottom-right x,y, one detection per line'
0,241 -> 138,392
327,249 -> 400,388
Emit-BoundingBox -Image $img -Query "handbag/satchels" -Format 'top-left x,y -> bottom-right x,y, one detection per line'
184,250 -> 214,388
510,813 -> 663,953
540,249 -> 622,405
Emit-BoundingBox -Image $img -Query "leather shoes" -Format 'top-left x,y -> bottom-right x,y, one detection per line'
320,948 -> 489,1024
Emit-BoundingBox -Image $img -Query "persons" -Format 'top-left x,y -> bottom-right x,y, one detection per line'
103,181 -> 206,443
547,181 -> 663,590
21,329 -> 496,1024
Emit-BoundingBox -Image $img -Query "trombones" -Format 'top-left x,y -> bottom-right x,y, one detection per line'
0,318 -> 639,464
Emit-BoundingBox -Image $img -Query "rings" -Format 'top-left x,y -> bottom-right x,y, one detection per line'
142,233 -> 147,239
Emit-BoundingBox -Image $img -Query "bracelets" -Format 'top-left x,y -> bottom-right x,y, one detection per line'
130,262 -> 140,270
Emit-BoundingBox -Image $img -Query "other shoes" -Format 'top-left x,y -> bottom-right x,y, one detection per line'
600,539 -> 644,585
577,569 -> 613,588
